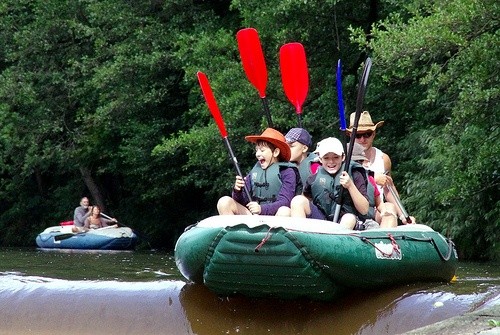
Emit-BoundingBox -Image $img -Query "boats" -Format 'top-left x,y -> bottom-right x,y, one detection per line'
174,214 -> 459,302
35,221 -> 138,251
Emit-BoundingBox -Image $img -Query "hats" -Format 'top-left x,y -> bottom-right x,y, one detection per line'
318,137 -> 345,159
346,143 -> 368,161
339,111 -> 384,132
284,127 -> 313,149
312,142 -> 321,154
244,127 -> 291,162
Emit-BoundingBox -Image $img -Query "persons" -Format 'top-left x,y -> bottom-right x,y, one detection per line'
285,128 -> 322,189
217,128 -> 296,218
339,111 -> 416,229
74,197 -> 117,231
290,136 -> 370,230
84,206 -> 108,232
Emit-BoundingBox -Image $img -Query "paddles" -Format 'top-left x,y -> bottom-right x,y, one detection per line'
100,213 -> 147,238
337,60 -> 347,161
54,224 -> 119,242
384,169 -> 456,281
279,42 -> 309,128
333,57 -> 372,223
236,28 -> 273,128
197,71 -> 252,202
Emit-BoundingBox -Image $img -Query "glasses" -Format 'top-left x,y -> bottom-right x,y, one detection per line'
355,131 -> 373,139
355,160 -> 364,162
